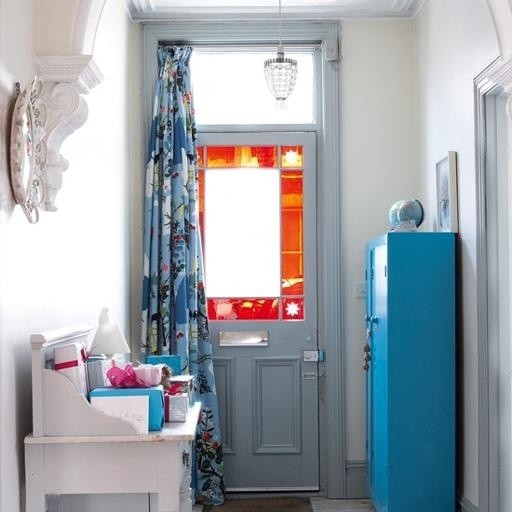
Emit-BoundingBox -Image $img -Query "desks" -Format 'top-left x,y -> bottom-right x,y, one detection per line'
23,323 -> 201,512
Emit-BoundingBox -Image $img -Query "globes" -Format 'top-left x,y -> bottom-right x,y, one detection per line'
388,199 -> 424,232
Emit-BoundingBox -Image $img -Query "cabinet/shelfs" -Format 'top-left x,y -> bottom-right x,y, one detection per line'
363,231 -> 456,512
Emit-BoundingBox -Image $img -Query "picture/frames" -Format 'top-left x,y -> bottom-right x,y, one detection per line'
432,151 -> 459,233
10,74 -> 49,224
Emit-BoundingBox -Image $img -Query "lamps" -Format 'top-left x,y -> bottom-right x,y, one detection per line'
262,0 -> 298,102
88,324 -> 132,358
